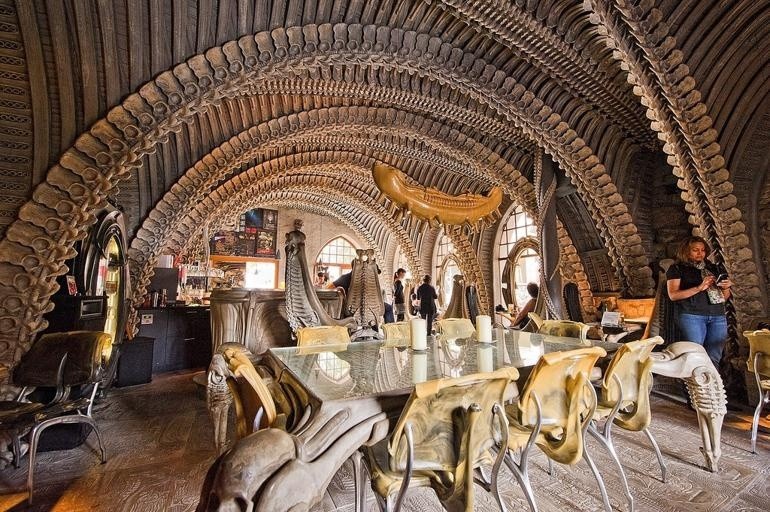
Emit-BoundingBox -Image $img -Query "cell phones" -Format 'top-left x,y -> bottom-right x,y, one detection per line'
716,274 -> 726,283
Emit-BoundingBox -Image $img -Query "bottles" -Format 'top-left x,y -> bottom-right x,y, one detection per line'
410,318 -> 427,350
144,289 -> 168,308
599,301 -> 624,325
476,315 -> 492,342
410,351 -> 427,384
477,344 -> 493,373
177,283 -> 204,305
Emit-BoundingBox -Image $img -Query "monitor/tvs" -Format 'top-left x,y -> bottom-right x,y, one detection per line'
148,268 -> 178,303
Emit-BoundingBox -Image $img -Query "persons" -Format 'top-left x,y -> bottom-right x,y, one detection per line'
509,282 -> 539,329
392,268 -> 407,322
326,259 -> 354,296
666,234 -> 732,411
315,272 -> 328,287
416,275 -> 438,336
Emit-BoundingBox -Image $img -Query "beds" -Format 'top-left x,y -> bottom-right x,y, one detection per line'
136,308 -> 212,373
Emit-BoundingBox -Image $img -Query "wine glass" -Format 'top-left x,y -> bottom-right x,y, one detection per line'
159,255 -> 224,277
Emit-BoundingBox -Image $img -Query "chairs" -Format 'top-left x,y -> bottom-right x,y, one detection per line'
0,331 -> 113,507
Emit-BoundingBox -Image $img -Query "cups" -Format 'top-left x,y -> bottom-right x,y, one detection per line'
203,293 -> 211,305
508,304 -> 514,313
